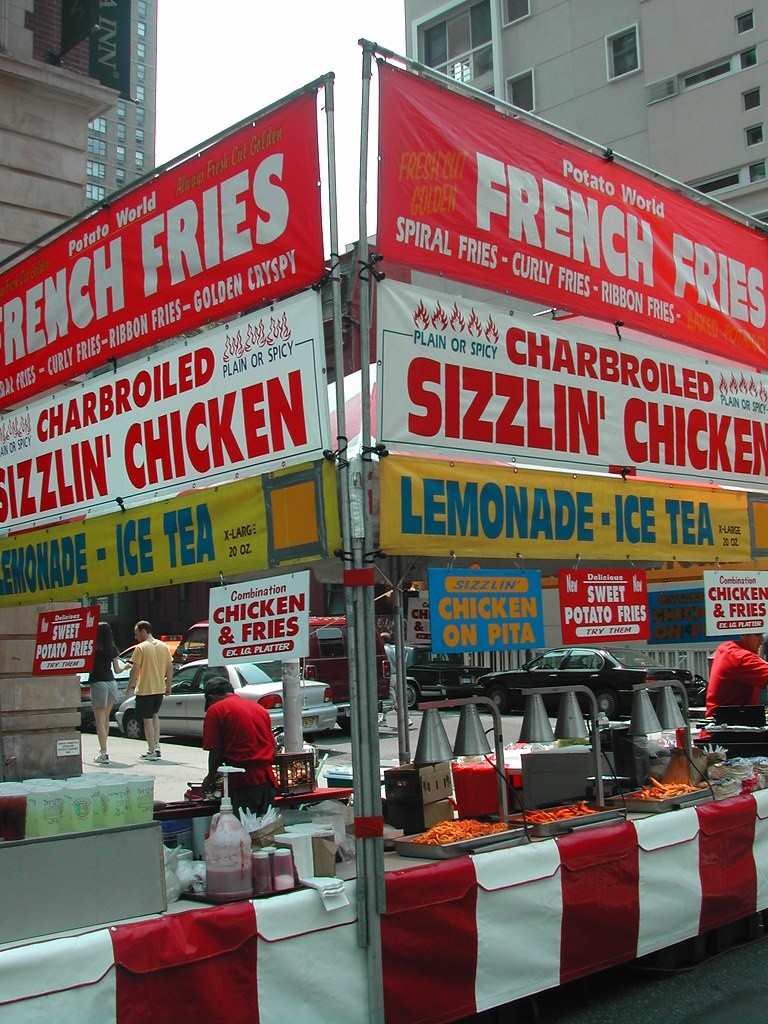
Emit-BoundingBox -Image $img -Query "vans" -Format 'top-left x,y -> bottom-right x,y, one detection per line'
172,616 -> 392,732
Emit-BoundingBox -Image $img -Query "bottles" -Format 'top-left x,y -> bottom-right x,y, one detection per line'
273,849 -> 295,891
261,847 -> 276,888
251,851 -> 272,894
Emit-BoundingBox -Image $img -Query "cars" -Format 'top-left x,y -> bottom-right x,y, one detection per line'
115,658 -> 337,749
75,634 -> 204,733
405,644 -> 493,709
473,644 -> 699,720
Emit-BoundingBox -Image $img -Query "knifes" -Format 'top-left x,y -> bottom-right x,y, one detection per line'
154,800 -> 197,811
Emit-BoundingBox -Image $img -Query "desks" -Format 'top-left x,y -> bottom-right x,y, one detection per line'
0,759 -> 768,1024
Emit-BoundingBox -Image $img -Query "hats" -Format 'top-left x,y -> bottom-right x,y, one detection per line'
204,677 -> 229,711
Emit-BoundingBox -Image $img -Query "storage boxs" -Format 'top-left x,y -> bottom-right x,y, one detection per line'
387,801 -> 453,833
384,762 -> 453,806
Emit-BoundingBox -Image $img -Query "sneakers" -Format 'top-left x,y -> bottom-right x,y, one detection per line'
138,749 -> 157,761
93,750 -> 109,764
154,743 -> 161,757
407,719 -> 413,726
378,721 -> 387,727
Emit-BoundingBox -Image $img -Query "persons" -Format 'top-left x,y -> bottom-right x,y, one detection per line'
700,633 -> 768,739
88,622 -> 133,764
201,676 -> 280,821
125,620 -> 173,761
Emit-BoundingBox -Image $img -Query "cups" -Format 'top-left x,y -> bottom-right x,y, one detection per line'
0,773 -> 156,842
